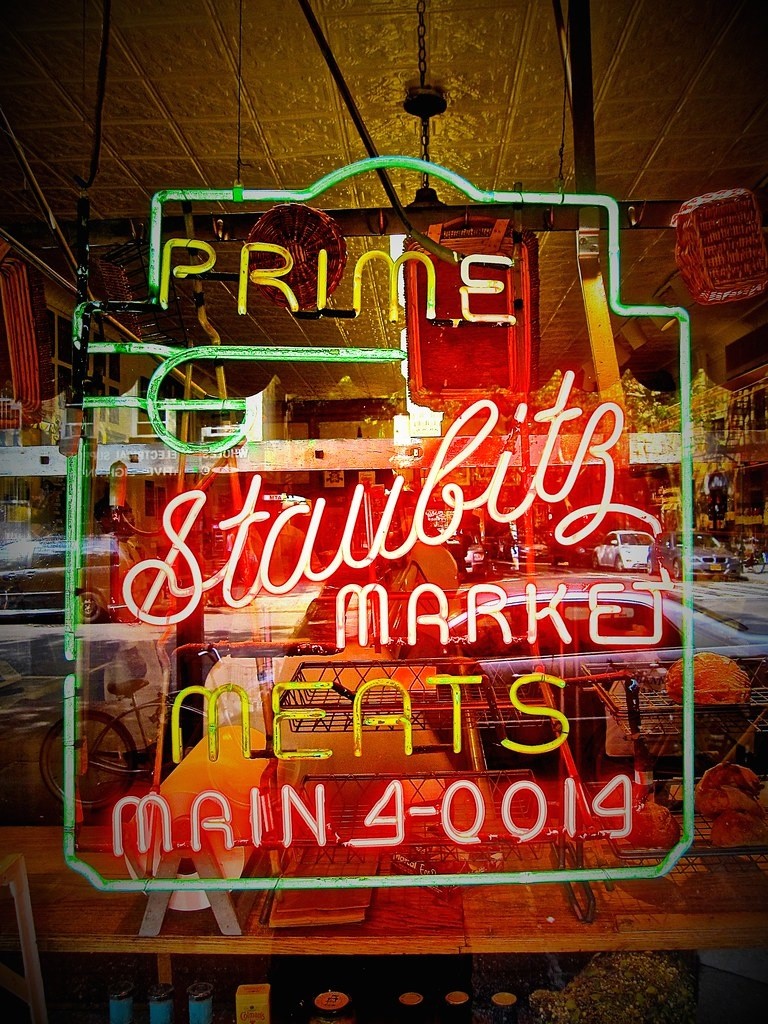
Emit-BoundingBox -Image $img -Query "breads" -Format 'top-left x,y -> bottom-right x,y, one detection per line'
610,761 -> 768,850
666,653 -> 752,705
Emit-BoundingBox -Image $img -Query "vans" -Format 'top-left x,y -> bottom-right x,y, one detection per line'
457,525 -> 485,574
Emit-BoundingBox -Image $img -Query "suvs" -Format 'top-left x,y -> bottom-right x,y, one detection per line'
0,534 -> 148,625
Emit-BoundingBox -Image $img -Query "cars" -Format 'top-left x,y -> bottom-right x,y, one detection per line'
386,574 -> 768,772
547,530 -> 606,567
647,531 -> 743,581
503,535 -> 550,561
592,530 -> 656,573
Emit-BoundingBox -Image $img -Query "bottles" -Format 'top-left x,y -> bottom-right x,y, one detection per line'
489,993 -> 517,1024
443,990 -> 473,1024
397,990 -> 427,1024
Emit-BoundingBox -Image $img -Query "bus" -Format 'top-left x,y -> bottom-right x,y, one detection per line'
654,486 -> 681,504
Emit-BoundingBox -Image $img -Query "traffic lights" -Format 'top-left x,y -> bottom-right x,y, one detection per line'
707,474 -> 728,519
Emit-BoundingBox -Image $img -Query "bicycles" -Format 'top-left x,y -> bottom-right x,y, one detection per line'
733,535 -> 767,574
39,643 -> 308,811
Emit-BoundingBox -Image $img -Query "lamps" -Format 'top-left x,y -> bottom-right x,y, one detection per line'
390,82 -> 447,308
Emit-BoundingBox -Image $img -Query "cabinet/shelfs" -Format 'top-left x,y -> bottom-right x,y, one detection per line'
0,363 -> 768,1024
